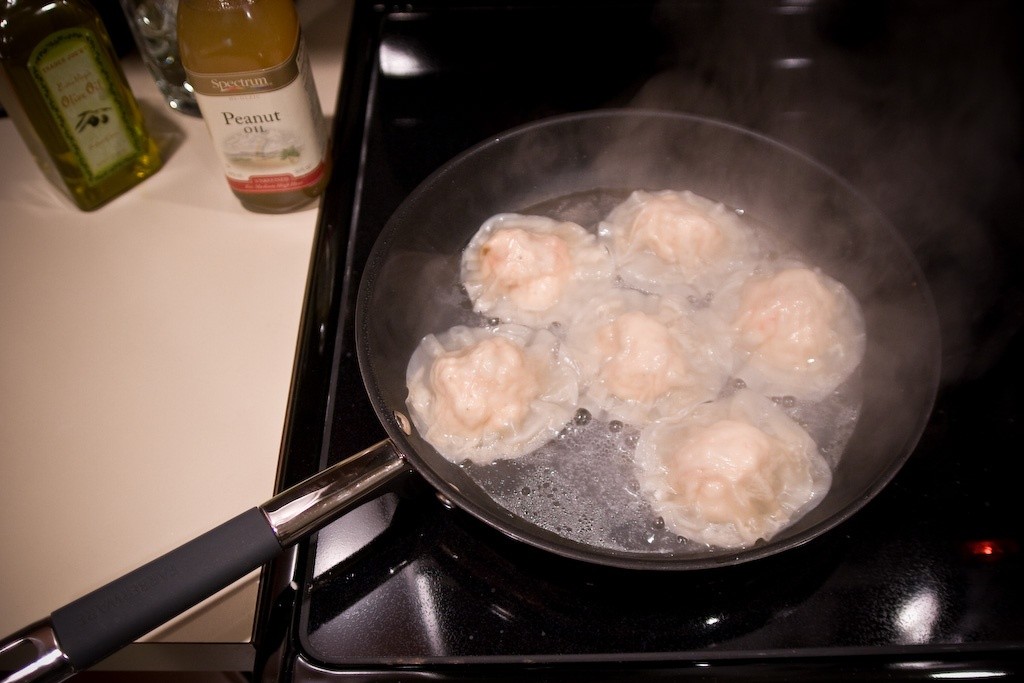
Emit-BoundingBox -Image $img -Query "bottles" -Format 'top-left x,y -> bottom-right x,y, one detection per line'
0,0 -> 162,210
121,0 -> 202,115
176,0 -> 329,215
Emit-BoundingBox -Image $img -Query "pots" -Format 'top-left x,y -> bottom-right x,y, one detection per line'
0,110 -> 941,683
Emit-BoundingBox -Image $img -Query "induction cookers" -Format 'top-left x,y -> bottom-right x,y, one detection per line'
253,0 -> 1024,683
